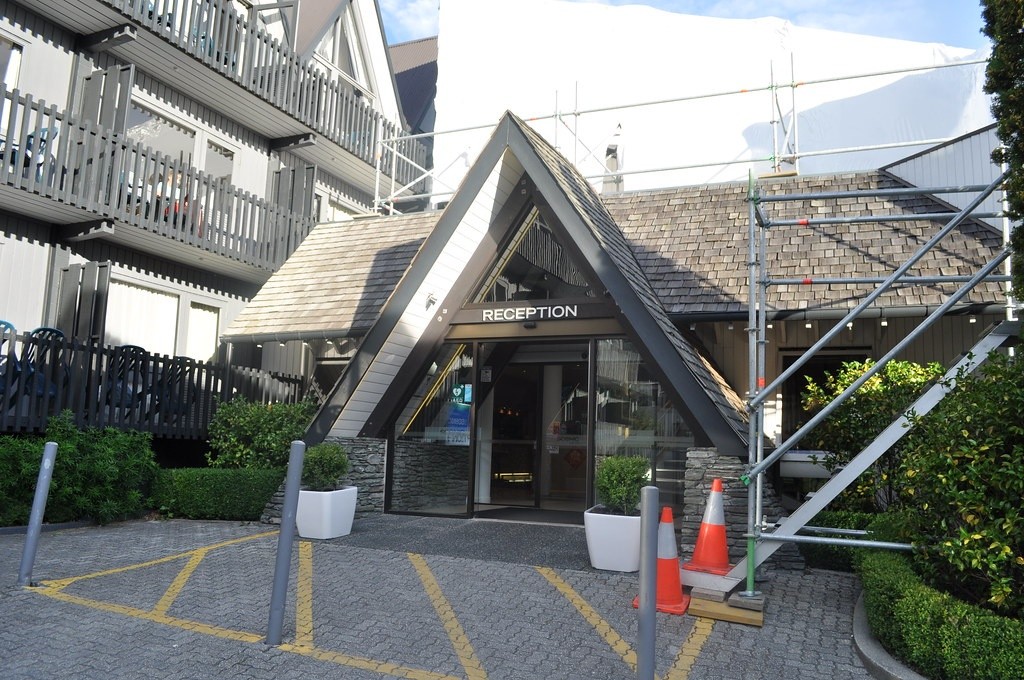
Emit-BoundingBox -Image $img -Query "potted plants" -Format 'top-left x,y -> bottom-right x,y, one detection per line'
584,455 -> 654,572
296,442 -> 357,540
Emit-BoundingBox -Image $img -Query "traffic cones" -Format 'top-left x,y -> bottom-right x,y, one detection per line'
682,478 -> 736,575
633,507 -> 691,615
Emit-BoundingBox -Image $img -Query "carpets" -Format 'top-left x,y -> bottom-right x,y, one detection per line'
294,506 -> 611,573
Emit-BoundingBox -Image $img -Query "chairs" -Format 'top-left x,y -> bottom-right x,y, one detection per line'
130,0 -> 235,66
0,127 -> 58,177
0,320 -> 196,429
